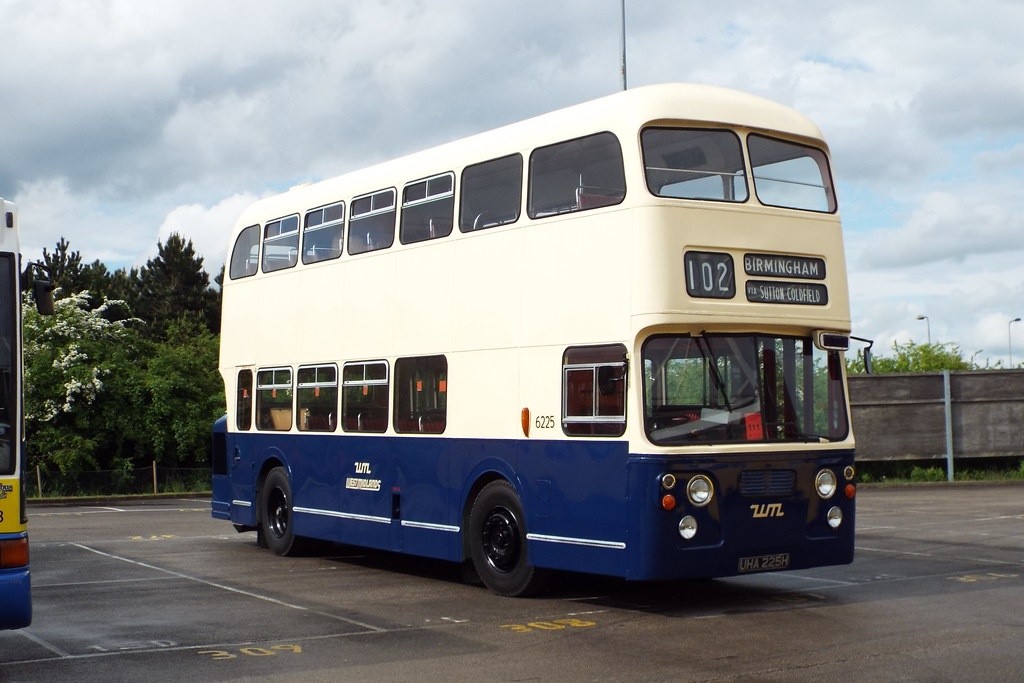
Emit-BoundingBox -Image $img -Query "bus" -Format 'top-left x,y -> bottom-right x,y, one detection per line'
211,83 -> 874,597
0,195 -> 56,631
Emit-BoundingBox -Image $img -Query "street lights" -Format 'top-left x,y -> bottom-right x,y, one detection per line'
1009,318 -> 1021,370
917,315 -> 930,345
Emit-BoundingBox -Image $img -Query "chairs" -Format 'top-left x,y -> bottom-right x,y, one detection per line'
578,404 -> 619,434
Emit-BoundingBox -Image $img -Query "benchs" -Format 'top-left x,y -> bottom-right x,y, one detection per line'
265,254 -> 294,272
245,254 -> 272,274
312,243 -> 340,261
429,217 -> 475,237
357,408 -> 418,432
575,186 -> 624,210
338,238 -> 368,254
303,407 -> 335,430
288,248 -> 314,266
472,210 -> 519,229
328,407 -> 358,430
367,230 -> 412,249
418,409 -> 445,432
